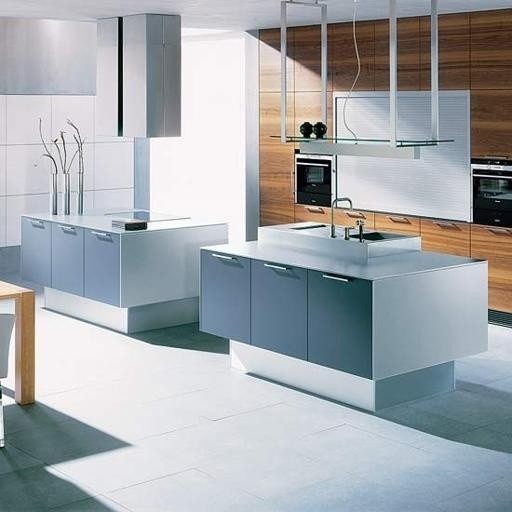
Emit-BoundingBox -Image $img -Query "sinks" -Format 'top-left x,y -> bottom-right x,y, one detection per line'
258,221 -> 423,257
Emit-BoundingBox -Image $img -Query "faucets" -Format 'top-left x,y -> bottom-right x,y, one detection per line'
329,198 -> 353,237
